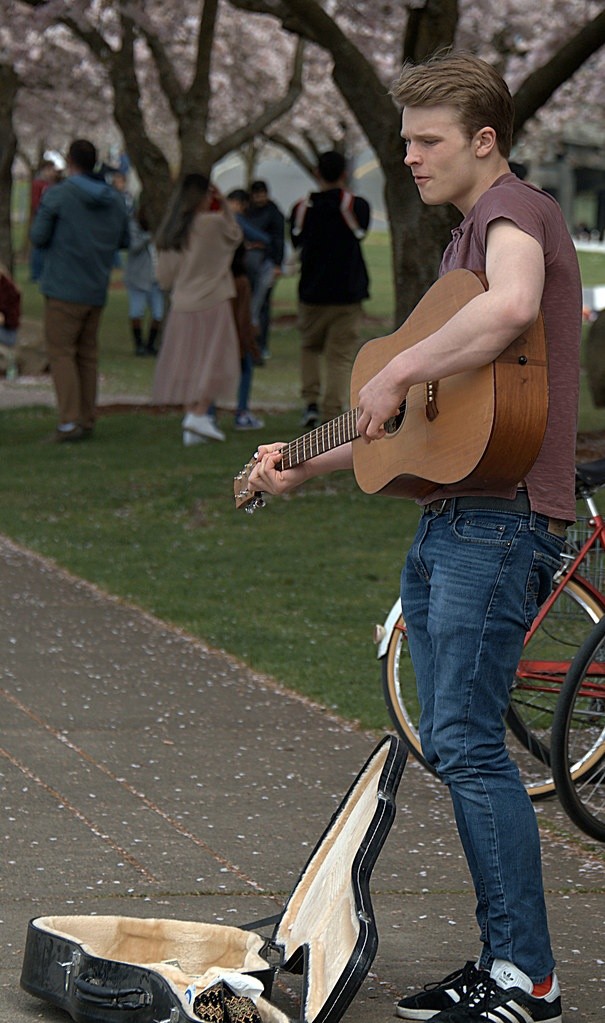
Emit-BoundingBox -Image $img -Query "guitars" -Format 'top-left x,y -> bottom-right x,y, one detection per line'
230,266 -> 550,512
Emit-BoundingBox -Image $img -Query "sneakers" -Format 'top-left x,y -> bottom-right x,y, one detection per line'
423,958 -> 563,1023
183,431 -> 212,446
396,960 -> 489,1021
181,413 -> 225,440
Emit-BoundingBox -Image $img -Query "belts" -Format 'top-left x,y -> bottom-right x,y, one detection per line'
424,490 -> 549,519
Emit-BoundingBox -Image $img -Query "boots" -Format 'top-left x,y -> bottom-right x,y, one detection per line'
129,317 -> 147,357
146,319 -> 162,356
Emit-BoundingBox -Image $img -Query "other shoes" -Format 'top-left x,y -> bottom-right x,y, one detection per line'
39,425 -> 85,443
301,403 -> 319,426
234,413 -> 265,431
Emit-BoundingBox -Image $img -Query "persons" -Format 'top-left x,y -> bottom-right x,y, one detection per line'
91,148 -> 167,358
155,173 -> 242,447
28,161 -> 67,284
30,141 -> 131,443
206,181 -> 284,432
288,152 -> 372,431
246,52 -> 581,1023
0,262 -> 22,374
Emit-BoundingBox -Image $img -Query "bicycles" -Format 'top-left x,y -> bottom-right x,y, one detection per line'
372,455 -> 605,839
